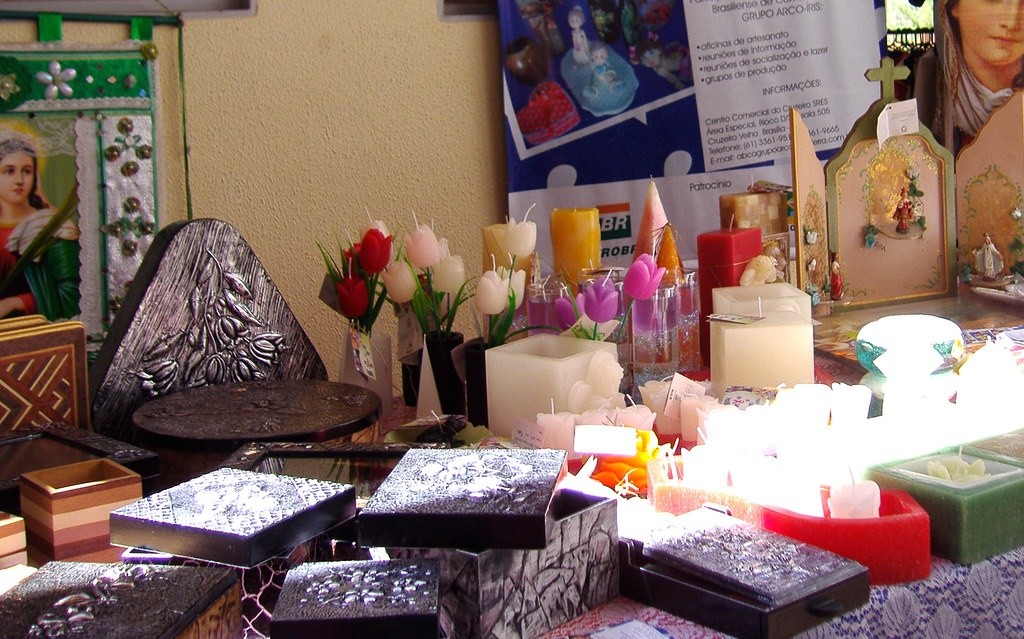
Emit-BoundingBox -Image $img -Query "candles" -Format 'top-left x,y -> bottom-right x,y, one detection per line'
711,282 -> 813,325
330,200 -> 668,343
709,294 -> 814,400
697,211 -> 764,370
533,375 -> 720,456
718,171 -> 789,282
925,445 -> 992,484
827,465 -> 882,521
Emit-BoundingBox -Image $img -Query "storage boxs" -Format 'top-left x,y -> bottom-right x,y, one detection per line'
18,457 -> 143,567
354,445 -> 619,639
110,466 -> 357,628
0,559 -> 243,639
